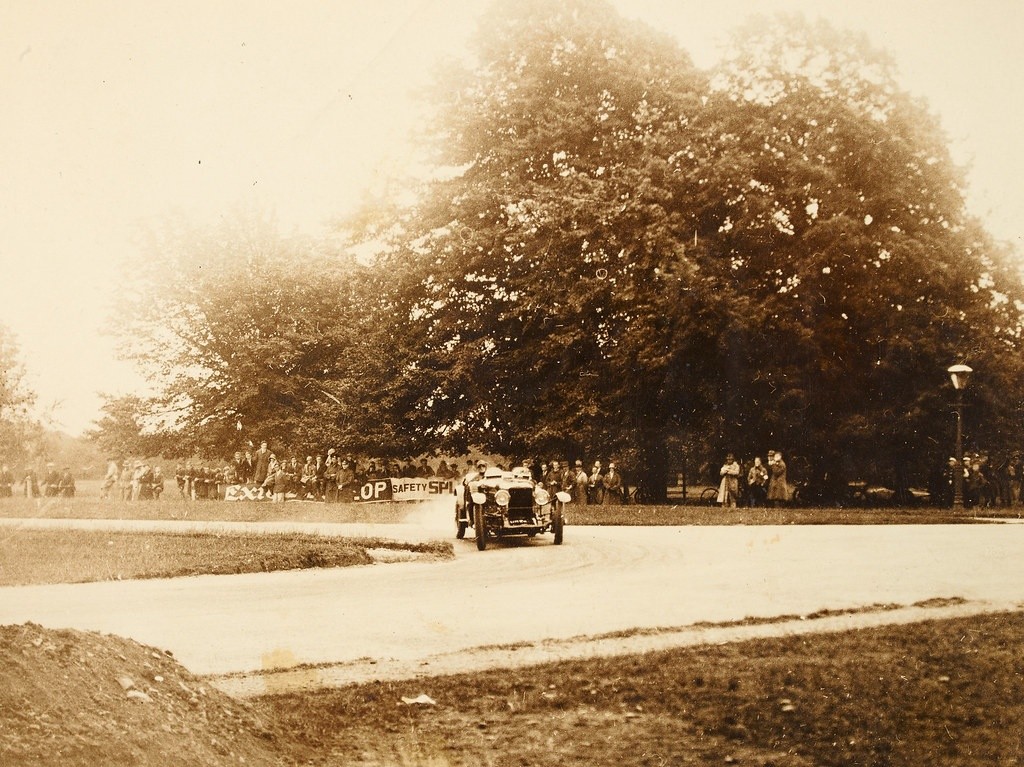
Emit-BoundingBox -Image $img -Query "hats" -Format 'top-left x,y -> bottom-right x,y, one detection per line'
268,454 -> 276,462
134,461 -> 142,468
346,454 -> 352,457
47,462 -> 53,466
726,454 -> 734,459
450,463 -> 458,467
143,463 -> 150,467
341,461 -> 347,465
609,463 -> 615,468
476,460 -> 487,467
63,467 -> 69,470
594,460 -> 602,467
122,461 -> 131,467
561,462 -> 568,466
767,450 -> 774,456
403,458 -> 412,462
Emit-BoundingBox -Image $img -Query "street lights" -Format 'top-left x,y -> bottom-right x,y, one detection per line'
945,365 -> 975,512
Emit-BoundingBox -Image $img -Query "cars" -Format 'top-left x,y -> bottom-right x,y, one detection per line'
452,470 -> 569,551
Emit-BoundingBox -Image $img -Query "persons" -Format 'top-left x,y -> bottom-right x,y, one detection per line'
0,441 -> 626,508
470,460 -> 490,481
719,450 -> 788,508
943,458 -> 1020,506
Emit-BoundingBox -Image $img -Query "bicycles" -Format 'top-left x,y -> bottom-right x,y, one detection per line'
621,476 -> 656,506
698,477 -> 726,507
